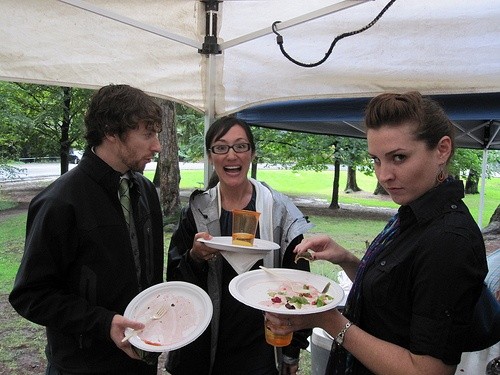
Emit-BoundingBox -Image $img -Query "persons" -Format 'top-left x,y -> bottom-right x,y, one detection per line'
263,90 -> 499,375
8,84 -> 164,375
165,116 -> 314,375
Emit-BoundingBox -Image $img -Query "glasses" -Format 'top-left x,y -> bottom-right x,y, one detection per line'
208,142 -> 252,154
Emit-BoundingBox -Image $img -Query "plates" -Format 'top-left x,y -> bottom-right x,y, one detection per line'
196,235 -> 281,255
228,268 -> 345,315
123,281 -> 213,352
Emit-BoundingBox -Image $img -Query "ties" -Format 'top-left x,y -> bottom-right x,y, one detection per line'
118,178 -> 142,292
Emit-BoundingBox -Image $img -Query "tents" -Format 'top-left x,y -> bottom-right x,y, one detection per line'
0,0 -> 500,150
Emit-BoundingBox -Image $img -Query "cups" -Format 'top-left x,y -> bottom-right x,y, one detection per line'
232,208 -> 261,247
265,312 -> 293,347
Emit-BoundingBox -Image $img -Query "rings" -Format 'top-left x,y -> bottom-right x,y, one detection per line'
288,318 -> 292,326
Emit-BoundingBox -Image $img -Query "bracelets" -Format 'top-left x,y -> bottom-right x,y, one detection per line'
334,319 -> 353,347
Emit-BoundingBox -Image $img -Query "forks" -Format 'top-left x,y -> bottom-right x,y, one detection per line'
260,265 -> 310,294
121,305 -> 168,342
